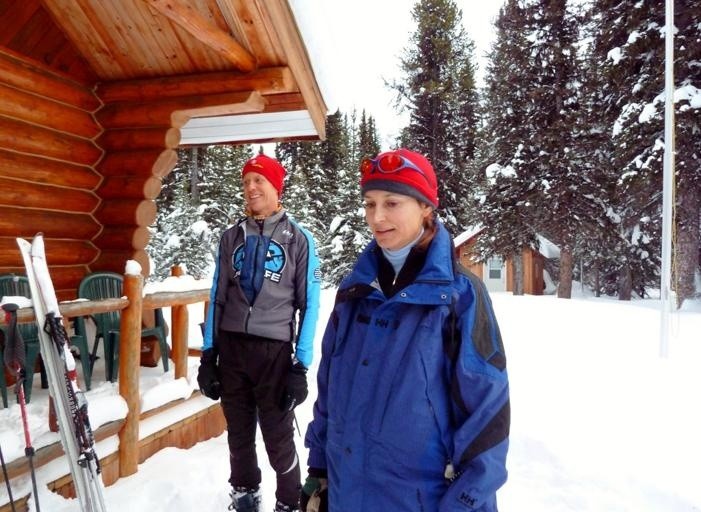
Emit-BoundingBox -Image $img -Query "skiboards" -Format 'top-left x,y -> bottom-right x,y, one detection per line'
16,230 -> 107,512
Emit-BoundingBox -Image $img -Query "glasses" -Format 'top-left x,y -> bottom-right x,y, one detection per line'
360,152 -> 428,181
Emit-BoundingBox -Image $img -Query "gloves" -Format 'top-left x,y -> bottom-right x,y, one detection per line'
197,348 -> 224,400
283,361 -> 309,411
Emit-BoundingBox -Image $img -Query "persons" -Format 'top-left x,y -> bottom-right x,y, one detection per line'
299,146 -> 510,511
196,154 -> 321,512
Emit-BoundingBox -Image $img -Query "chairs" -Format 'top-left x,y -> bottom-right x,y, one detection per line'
0,270 -> 94,407
78,270 -> 172,384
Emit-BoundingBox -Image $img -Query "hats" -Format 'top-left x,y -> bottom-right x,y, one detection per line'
360,149 -> 439,210
242,154 -> 287,199
